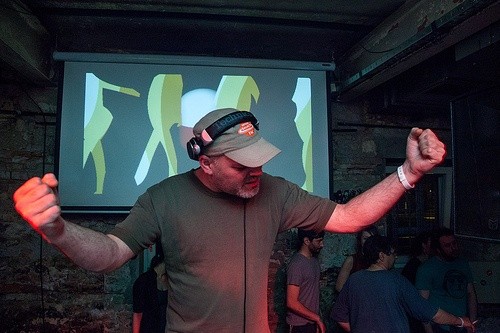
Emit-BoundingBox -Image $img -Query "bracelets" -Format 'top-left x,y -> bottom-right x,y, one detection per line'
458,317 -> 465,327
397,165 -> 415,190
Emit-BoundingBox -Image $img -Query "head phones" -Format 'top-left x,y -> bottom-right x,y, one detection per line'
186,111 -> 260,161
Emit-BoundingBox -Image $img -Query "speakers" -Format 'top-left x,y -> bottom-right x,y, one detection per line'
450,82 -> 500,243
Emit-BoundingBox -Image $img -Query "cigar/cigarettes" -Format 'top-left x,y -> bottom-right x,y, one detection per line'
472,320 -> 478,325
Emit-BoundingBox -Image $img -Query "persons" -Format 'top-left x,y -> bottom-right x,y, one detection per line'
131,239 -> 169,333
401,226 -> 477,333
13,107 -> 446,333
335,225 -> 379,292
329,234 -> 477,333
284,228 -> 326,333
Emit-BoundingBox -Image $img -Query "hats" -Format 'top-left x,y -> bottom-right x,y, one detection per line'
193,108 -> 282,167
